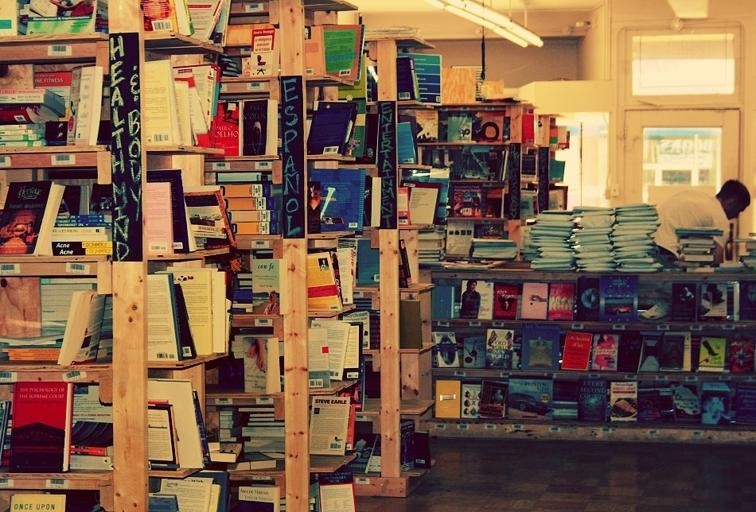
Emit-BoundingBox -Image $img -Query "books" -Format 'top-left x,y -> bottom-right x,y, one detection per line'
572,204 -> 756,430
0,2 -> 570,512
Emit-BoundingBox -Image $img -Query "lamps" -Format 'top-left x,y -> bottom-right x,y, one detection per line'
429,0 -> 544,48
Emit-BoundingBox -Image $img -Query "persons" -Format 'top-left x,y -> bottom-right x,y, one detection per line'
649,177 -> 752,268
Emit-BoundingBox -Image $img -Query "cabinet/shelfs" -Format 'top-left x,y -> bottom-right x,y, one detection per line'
0,32 -> 571,512
417,261 -> 755,444
